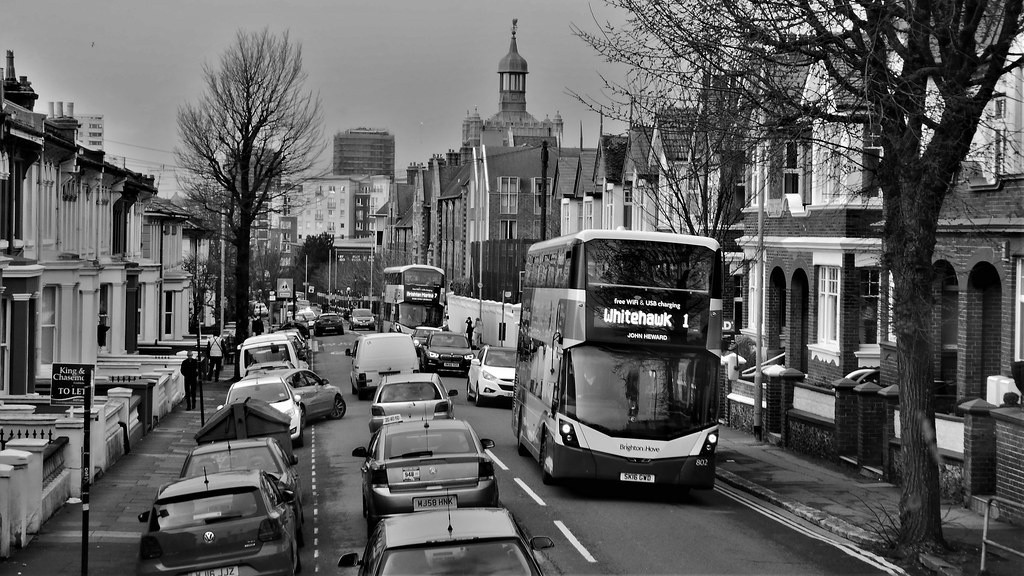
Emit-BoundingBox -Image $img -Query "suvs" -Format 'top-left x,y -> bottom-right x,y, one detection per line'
466,345 -> 519,406
313,313 -> 344,337
217,358 -> 346,445
137,466 -> 303,576
348,308 -> 376,330
416,331 -> 476,378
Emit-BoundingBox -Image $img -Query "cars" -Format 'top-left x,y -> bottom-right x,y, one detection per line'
178,437 -> 304,511
338,486 -> 555,576
368,373 -> 458,434
237,291 -> 317,380
352,403 -> 499,538
411,326 -> 443,355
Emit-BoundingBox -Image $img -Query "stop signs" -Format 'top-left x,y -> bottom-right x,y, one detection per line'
478,282 -> 483,288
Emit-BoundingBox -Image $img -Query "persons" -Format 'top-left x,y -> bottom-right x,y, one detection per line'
465,317 -> 482,349
225,331 -> 235,364
207,330 -> 227,382
252,315 -> 264,336
181,351 -> 198,410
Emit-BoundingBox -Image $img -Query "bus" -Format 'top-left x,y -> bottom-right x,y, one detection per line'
511,226 -> 738,490
383,263 -> 449,336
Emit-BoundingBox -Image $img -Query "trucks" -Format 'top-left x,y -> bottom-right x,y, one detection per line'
345,332 -> 420,400
